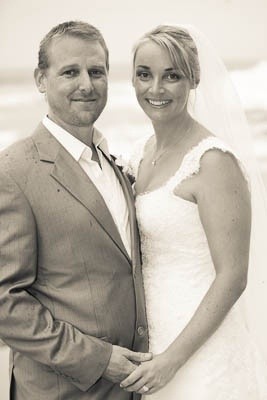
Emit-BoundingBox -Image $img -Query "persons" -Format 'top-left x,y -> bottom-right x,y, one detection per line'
0,21 -> 150,400
119,23 -> 254,400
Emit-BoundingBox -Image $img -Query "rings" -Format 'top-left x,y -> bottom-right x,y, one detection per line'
142,385 -> 149,392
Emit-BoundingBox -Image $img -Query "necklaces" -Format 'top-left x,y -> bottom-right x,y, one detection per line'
149,119 -> 194,167
